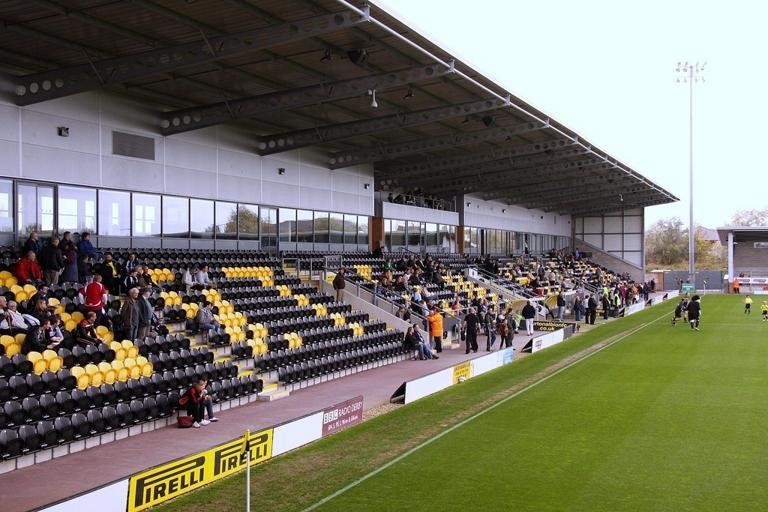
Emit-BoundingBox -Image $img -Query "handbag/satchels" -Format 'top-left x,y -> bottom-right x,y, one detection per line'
177,415 -> 193,428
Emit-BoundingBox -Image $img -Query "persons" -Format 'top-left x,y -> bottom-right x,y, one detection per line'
367,239 -> 656,359
332,267 -> 346,304
761,300 -> 768,321
178,380 -> 205,428
733,278 -> 739,294
0,231 -> 222,356
739,272 -> 744,277
671,294 -> 702,331
199,376 -> 219,423
388,190 -> 446,210
744,295 -> 752,314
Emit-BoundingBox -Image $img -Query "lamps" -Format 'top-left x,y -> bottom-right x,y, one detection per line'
345,48 -> 368,67
483,115 -> 497,128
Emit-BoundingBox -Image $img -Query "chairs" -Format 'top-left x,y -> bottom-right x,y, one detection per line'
0,243 -> 647,473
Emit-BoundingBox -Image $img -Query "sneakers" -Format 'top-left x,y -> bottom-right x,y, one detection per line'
193,416 -> 219,428
